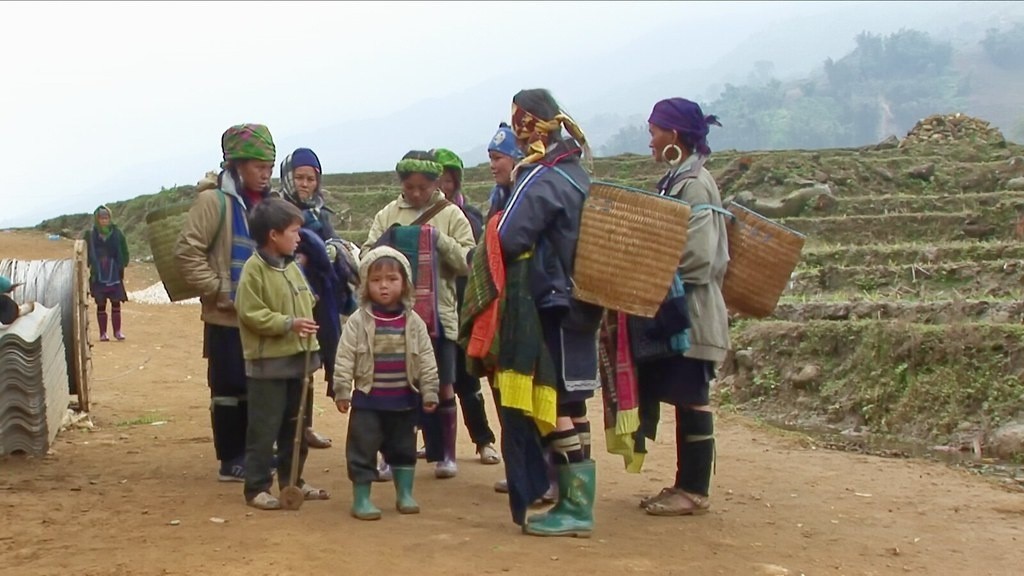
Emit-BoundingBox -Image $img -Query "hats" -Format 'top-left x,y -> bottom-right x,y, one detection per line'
97,208 -> 110,217
291,149 -> 320,169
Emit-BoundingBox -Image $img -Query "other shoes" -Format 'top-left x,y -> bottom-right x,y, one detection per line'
478,441 -> 500,463
218,456 -> 276,482
416,445 -> 427,458
303,427 -> 332,448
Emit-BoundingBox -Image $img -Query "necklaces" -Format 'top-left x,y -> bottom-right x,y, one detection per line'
365,306 -> 406,321
255,248 -> 294,271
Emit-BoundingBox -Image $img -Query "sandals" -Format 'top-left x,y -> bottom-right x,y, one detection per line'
646,487 -> 710,515
641,485 -> 678,508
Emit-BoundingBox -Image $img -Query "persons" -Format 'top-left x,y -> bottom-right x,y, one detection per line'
332,245 -> 439,519
87,205 -> 129,341
487,89 -> 600,537
359,148 -> 500,480
640,97 -> 730,515
175,123 -> 340,509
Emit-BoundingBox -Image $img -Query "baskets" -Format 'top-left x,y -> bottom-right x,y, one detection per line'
570,182 -> 690,319
146,200 -> 205,302
720,200 -> 806,318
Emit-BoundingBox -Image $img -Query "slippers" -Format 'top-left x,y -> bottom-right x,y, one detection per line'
300,483 -> 330,500
246,492 -> 283,510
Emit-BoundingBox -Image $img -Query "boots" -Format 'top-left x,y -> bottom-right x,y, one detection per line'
351,479 -> 382,519
391,466 -> 420,513
526,458 -> 596,537
532,445 -> 559,504
375,455 -> 392,482
495,479 -> 509,492
434,405 -> 457,478
111,312 -> 125,340
96,313 -> 110,341
